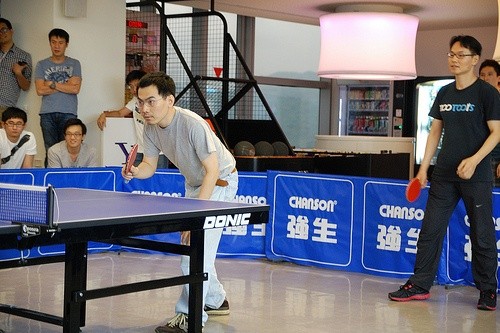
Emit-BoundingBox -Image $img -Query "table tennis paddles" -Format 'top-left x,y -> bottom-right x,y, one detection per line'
124,143 -> 138,184
405,177 -> 428,203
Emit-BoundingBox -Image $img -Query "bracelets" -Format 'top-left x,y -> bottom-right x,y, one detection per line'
103,111 -> 111,117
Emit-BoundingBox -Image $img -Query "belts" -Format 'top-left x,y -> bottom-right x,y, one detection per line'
216,168 -> 236,186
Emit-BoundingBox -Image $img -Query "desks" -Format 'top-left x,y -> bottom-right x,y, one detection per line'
233,154 -> 355,173
0,183 -> 270,333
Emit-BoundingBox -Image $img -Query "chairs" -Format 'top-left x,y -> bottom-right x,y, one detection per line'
272,142 -> 289,157
233,141 -> 255,156
254,141 -> 274,156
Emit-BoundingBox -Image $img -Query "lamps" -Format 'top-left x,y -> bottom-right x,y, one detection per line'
316,3 -> 421,81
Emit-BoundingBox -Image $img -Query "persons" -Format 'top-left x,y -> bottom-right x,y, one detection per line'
97,70 -> 168,168
388,34 -> 500,309
47,119 -> 97,168
34,29 -> 82,167
0,107 -> 37,168
0,18 -> 32,120
120,73 -> 238,333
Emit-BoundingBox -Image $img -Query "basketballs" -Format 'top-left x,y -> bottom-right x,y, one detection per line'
233,140 -> 255,157
272,142 -> 289,156
254,141 -> 275,156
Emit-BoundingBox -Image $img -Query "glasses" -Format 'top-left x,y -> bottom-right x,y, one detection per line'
64,132 -> 81,137
128,84 -> 137,89
135,97 -> 165,108
0,28 -> 9,33
447,51 -> 474,59
4,121 -> 23,128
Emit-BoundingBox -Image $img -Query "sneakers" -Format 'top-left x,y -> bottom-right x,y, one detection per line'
477,292 -> 497,310
154,313 -> 189,333
388,280 -> 430,301
204,299 -> 230,315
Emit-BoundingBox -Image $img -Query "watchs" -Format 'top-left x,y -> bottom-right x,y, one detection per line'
50,81 -> 56,89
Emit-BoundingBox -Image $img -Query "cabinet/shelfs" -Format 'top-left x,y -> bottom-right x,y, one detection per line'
345,84 -> 390,137
